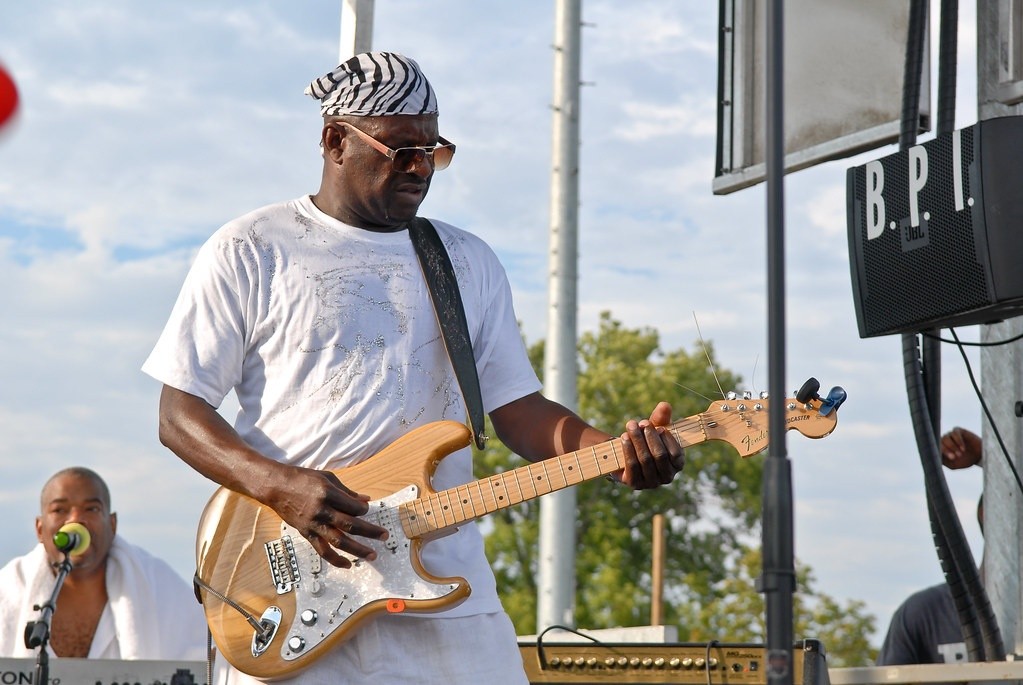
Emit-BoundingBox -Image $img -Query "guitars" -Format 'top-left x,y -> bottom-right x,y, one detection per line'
193,380 -> 848,682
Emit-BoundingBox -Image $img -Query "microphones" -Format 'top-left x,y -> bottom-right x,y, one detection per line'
795,378 -> 832,405
52,522 -> 91,555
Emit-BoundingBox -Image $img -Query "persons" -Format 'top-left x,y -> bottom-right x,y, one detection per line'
878,426 -> 984,667
0,467 -> 217,661
141,51 -> 684,685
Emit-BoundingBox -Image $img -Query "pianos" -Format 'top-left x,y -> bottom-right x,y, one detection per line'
0,657 -> 212,685
507,635 -> 833,684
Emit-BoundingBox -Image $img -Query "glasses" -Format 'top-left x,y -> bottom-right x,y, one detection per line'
320,122 -> 456,174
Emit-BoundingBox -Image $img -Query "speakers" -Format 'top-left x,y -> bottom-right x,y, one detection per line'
847,115 -> 1023,339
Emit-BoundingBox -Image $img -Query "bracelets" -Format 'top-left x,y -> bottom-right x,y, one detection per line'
602,437 -> 619,482
978,456 -> 984,468
